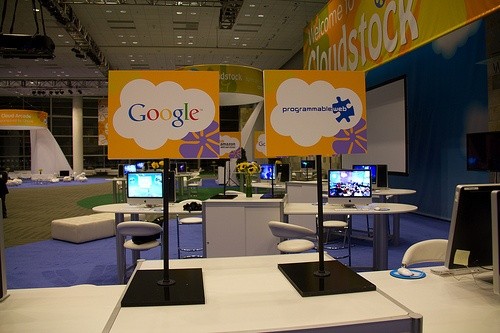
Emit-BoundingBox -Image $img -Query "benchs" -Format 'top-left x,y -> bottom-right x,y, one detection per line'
51,212 -> 146,244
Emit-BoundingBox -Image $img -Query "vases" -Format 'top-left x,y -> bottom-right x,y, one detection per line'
246,181 -> 251,196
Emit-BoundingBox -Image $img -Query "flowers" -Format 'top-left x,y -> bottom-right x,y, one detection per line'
235,161 -> 260,186
151,161 -> 164,172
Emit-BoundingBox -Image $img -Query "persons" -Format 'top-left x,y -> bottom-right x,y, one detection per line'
0,167 -> 9,218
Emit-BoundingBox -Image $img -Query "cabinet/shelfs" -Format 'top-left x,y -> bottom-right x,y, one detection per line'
285,181 -> 318,203
202,193 -> 288,258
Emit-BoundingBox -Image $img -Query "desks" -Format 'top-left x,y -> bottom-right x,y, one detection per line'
0,284 -> 127,333
284,202 -> 418,270
355,266 -> 500,333
175,174 -> 190,196
244,183 -> 286,193
92,203 -> 202,285
102,250 -> 423,332
323,184 -> 417,246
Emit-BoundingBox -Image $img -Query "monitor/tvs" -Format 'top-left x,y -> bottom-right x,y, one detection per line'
301,160 -> 313,168
444,183 -> 500,269
466,130 -> 500,172
376,164 -> 388,189
127,171 -> 163,198
259,164 -> 275,180
177,162 -> 187,174
60,171 -> 69,176
276,164 -> 289,182
352,164 -> 378,189
328,169 -> 372,211
491,190 -> 500,296
137,163 -> 144,168
123,165 -> 137,174
168,171 -> 177,202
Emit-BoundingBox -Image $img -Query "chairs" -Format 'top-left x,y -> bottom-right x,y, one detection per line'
117,173 -> 449,284
